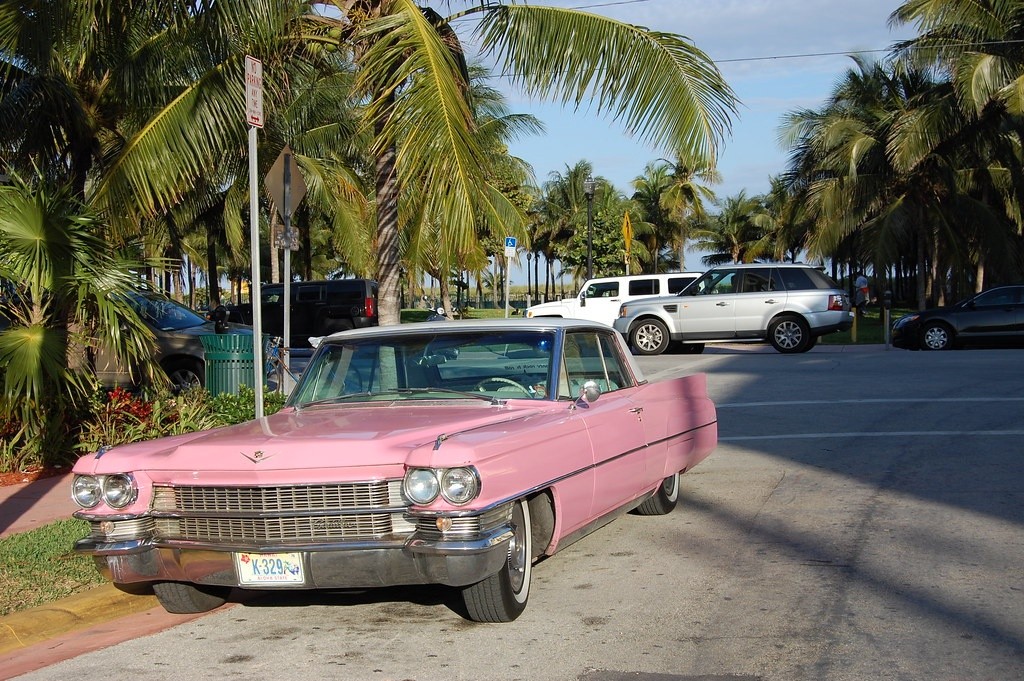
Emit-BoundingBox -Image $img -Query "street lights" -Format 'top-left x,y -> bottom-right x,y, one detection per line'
584,178 -> 597,283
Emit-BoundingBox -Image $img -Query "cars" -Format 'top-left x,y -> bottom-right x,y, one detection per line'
891,286 -> 1024,351
89,290 -> 280,400
400,309 -> 459,362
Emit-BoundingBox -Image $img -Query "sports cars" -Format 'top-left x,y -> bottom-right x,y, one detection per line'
70,317 -> 721,623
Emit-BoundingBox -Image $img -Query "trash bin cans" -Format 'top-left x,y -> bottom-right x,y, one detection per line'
198,333 -> 269,399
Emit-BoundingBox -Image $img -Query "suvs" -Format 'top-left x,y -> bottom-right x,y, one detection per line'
209,278 -> 381,345
524,272 -> 714,355
613,263 -> 854,354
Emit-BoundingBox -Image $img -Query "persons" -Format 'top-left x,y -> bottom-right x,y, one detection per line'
853,272 -> 869,314
203,294 -> 229,333
